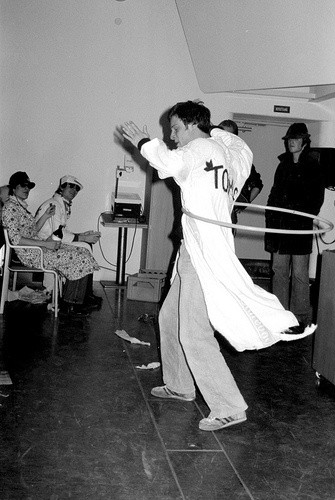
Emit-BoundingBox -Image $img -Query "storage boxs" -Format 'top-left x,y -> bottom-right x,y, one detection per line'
127,273 -> 161,302
112,192 -> 142,218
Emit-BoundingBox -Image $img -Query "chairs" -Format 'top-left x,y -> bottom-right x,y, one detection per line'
0,229 -> 63,317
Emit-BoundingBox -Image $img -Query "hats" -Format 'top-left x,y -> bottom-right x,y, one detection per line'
58,175 -> 84,191
9,172 -> 35,189
282,123 -> 311,139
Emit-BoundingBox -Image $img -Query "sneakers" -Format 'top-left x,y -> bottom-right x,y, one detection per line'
199,410 -> 247,431
151,385 -> 196,401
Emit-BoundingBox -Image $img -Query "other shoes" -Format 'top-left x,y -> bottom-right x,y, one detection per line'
82,294 -> 103,309
283,321 -> 306,334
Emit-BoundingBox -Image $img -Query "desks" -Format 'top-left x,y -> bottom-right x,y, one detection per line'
100,213 -> 149,287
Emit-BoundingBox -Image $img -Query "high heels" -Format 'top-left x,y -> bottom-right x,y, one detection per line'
58,302 -> 89,317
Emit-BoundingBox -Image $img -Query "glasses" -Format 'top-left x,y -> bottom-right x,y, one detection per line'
68,183 -> 81,191
20,182 -> 34,189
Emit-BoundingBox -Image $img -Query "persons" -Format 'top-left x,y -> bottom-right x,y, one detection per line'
121,100 -> 254,431
35,175 -> 103,310
216,119 -> 263,239
264,123 -> 325,329
1,171 -> 91,318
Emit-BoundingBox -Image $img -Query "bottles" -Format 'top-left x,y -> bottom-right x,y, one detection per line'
51,225 -> 63,252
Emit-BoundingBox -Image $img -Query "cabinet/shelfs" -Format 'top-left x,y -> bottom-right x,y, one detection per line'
313,250 -> 335,386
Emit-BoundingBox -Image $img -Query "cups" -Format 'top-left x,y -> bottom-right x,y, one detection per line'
102,212 -> 115,223
